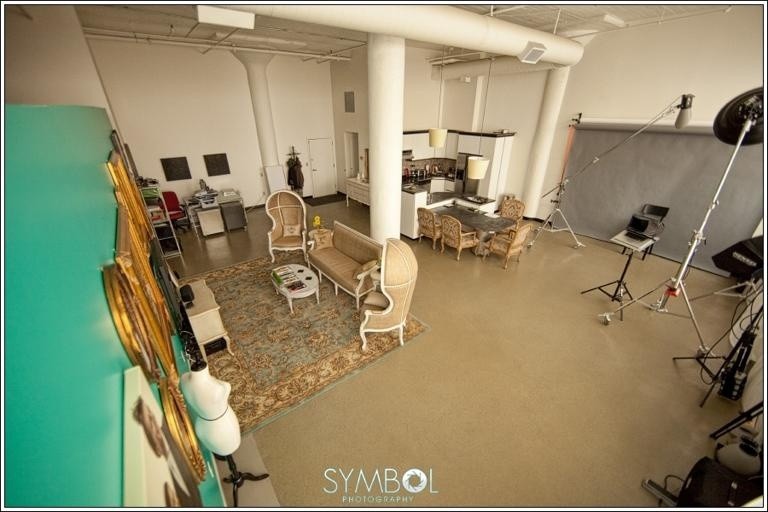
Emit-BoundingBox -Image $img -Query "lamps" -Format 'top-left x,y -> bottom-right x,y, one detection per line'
468,156 -> 489,179
529,92 -> 694,251
429,128 -> 447,147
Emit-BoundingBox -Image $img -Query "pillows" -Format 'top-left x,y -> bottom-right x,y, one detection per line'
353,260 -> 378,279
315,231 -> 334,250
365,291 -> 388,308
283,224 -> 302,237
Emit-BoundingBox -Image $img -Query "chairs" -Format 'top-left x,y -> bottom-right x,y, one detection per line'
265,189 -> 307,262
483,223 -> 532,270
502,201 -> 524,228
418,208 -> 443,250
440,218 -> 479,261
359,239 -> 419,351
160,191 -> 187,233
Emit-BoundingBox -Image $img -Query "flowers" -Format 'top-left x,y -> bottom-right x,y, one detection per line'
313,216 -> 322,231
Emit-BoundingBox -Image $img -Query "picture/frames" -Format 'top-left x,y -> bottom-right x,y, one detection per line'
159,377 -> 206,485
123,364 -> 205,508
105,264 -> 158,383
112,129 -> 136,177
117,203 -> 181,382
114,184 -> 169,302
106,150 -> 154,257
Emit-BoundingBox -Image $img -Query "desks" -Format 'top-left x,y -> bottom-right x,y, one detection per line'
184,187 -> 247,237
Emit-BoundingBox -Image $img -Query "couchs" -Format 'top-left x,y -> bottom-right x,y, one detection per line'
307,220 -> 383,314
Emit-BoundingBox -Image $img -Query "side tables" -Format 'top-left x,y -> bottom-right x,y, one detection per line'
309,228 -> 331,239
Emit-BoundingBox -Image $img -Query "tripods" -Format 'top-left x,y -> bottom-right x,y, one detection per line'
580,250 -> 636,321
672,303 -> 764,408
596,113 -> 750,353
527,104 -> 680,249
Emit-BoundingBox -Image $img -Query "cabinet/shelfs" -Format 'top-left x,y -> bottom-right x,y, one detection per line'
177,278 -> 234,363
401,182 -> 455,240
346,176 -> 370,208
139,181 -> 186,267
420,172 -> 456,193
